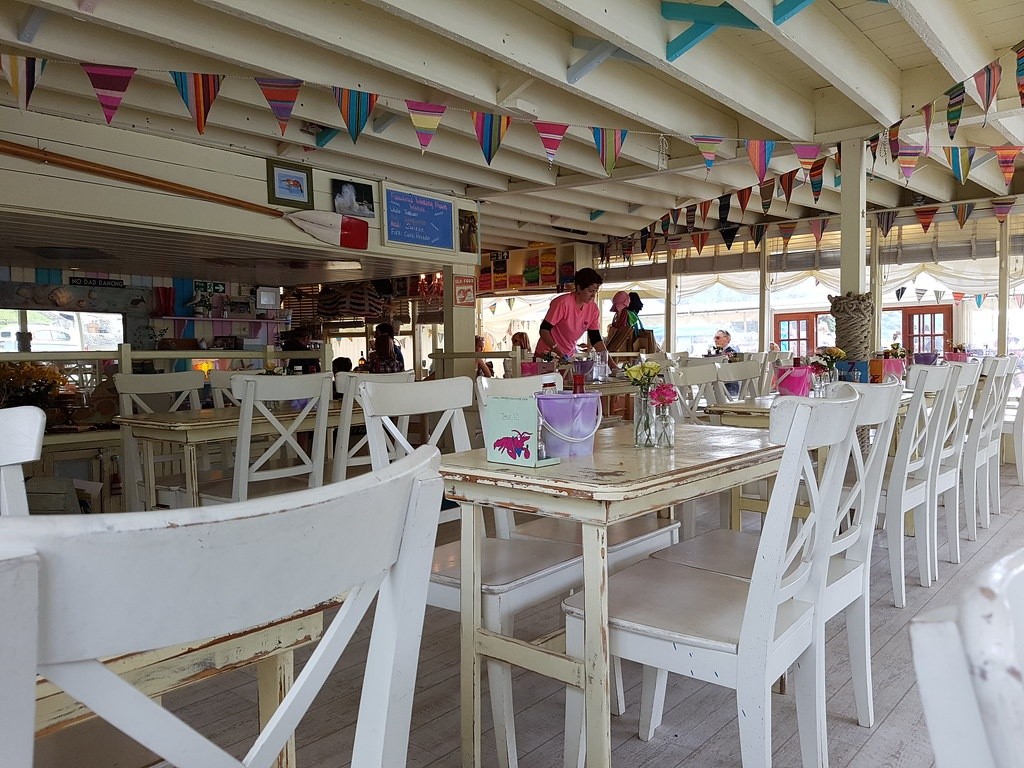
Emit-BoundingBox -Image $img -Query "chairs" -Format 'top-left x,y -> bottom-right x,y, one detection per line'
0,351 -> 1024,768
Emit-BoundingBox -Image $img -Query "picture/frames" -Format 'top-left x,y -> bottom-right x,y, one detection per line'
257,287 -> 280,309
224,295 -> 256,319
266,157 -> 314,210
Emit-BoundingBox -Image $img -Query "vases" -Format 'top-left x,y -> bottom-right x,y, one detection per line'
655,403 -> 677,449
823,362 -> 838,398
633,385 -> 656,447
814,379 -> 826,398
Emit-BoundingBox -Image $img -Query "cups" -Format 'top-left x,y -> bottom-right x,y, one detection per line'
592,351 -> 608,384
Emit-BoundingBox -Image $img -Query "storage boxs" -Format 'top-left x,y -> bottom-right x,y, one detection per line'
236,344 -> 263,352
237,336 -> 261,345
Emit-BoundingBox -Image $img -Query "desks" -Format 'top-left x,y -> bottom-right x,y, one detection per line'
441,401 -> 484,453
440,424 -> 830,768
564,376 -> 637,417
111,400 -> 366,508
888,379 -> 1007,466
704,392 -> 916,538
944,352 -> 972,363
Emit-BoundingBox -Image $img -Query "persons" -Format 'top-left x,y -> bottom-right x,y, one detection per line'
713,330 -> 740,396
287,329 -> 322,375
354,335 -> 404,373
475,335 -> 492,377
534,268 -> 623,375
512,332 -> 539,377
374,322 -> 404,370
333,357 -> 353,401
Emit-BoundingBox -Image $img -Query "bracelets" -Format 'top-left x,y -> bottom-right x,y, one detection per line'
551,343 -> 559,351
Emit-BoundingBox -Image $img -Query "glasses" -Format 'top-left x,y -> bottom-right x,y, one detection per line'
714,336 -> 727,340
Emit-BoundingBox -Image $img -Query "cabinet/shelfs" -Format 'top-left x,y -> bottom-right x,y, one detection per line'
476,241 -> 594,292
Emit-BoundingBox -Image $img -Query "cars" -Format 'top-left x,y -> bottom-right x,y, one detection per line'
1,325 -> 81,351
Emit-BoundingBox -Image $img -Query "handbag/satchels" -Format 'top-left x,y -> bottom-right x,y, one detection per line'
632,309 -> 657,354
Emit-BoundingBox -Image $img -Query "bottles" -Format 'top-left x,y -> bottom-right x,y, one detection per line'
573,375 -> 587,394
884,351 -> 890,359
293,366 -> 303,375
793,358 -> 801,368
358,350 -> 366,365
542,376 -> 558,395
952,348 -> 958,353
308,365 -> 316,374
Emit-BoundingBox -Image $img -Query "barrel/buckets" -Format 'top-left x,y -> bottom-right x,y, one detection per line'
884,359 -> 907,383
914,353 -> 939,366
945,352 -> 967,363
774,367 -> 811,397
573,360 -> 593,381
533,388 -> 603,458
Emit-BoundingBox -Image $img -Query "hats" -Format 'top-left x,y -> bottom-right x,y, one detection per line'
610,291 -> 630,311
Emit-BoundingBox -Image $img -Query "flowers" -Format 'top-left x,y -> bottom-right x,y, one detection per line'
815,346 -> 846,382
810,361 -> 829,397
624,361 -> 662,446
648,383 -> 680,447
881,342 -> 915,359
0,361 -> 68,409
